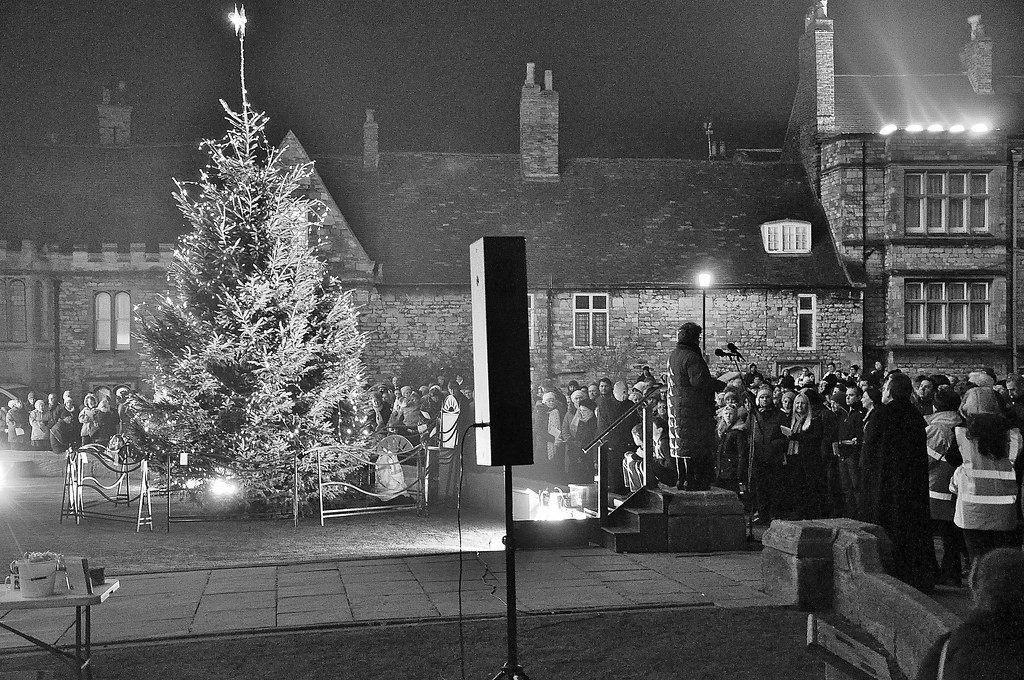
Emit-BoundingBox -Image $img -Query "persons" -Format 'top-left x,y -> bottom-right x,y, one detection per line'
1,361 -> 1023,592
666,322 -> 730,491
913,545 -> 1024,680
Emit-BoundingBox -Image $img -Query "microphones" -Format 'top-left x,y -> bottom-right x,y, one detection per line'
716,343 -> 746,362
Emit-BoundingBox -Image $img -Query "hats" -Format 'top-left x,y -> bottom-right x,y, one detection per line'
61,409 -> 72,419
781,391 -> 795,402
428,384 -> 441,392
756,389 -> 772,401
633,381 -> 649,391
400,385 -> 411,396
570,390 -> 588,401
540,392 -> 556,404
968,370 -> 994,385
960,387 -> 1003,416
578,399 -> 598,411
64,397 -> 73,405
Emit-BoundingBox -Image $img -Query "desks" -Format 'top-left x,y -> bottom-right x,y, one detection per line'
0,574 -> 120,680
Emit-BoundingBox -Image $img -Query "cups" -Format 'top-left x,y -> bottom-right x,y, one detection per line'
89,565 -> 104,587
5,575 -> 20,590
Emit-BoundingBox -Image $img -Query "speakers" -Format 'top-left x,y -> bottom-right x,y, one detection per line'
468,234 -> 534,467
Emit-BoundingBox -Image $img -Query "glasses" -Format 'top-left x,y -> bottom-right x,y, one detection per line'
699,336 -> 701,342
